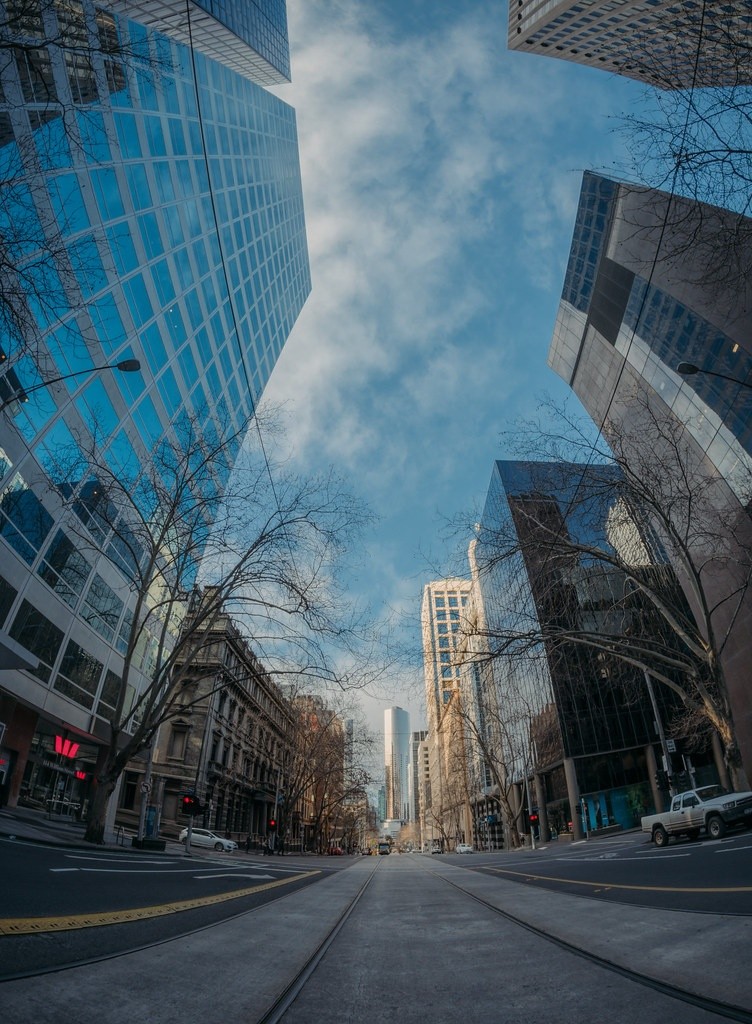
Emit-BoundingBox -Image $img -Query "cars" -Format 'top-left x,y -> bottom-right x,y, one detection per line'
455,844 -> 474,854
431,848 -> 441,854
179,827 -> 239,852
361,848 -> 372,855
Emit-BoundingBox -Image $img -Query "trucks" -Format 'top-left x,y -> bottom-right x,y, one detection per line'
378,842 -> 391,855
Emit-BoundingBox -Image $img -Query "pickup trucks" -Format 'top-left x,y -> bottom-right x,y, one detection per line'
641,784 -> 752,847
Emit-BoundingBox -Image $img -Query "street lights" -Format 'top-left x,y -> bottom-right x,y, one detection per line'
1,356 -> 142,408
675,360 -> 752,396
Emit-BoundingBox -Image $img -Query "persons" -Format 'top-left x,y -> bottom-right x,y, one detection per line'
224,829 -> 231,840
591,793 -> 603,828
277,839 -> 285,856
245,832 -> 251,853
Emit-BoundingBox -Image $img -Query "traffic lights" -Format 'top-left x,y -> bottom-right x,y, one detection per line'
529,814 -> 540,825
269,820 -> 277,830
180,796 -> 199,817
